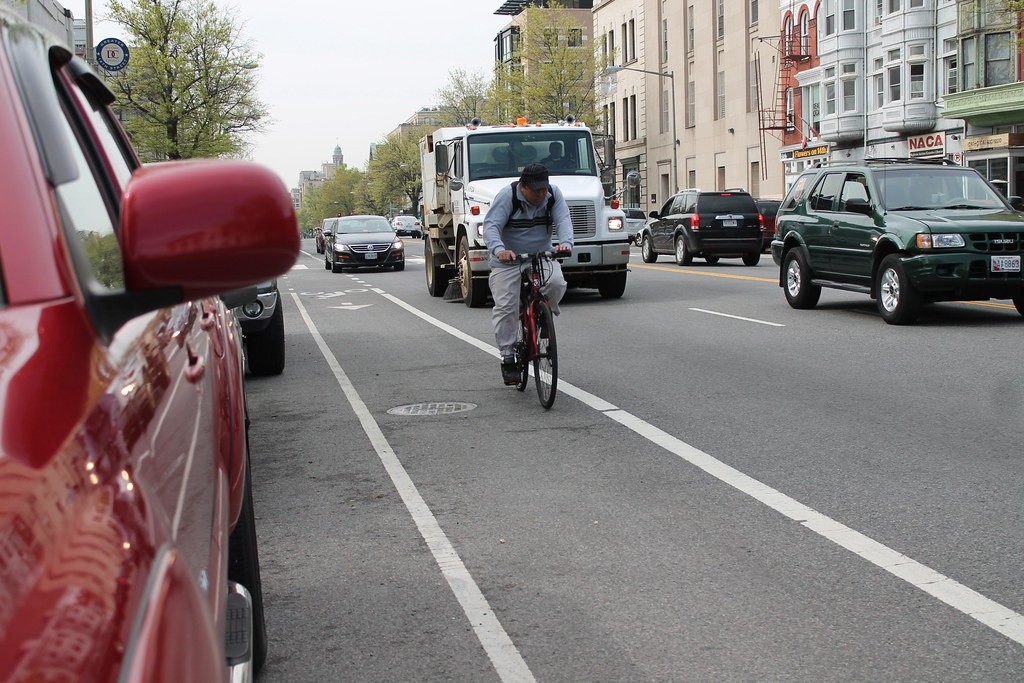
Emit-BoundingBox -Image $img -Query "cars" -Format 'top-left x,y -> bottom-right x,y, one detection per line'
395,214 -> 424,237
225,278 -> 287,382
315,214 -> 407,270
0,7 -> 301,683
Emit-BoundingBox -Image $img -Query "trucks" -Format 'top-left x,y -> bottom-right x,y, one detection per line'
420,116 -> 629,310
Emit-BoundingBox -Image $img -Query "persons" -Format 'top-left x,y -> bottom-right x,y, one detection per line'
483,163 -> 574,385
539,142 -> 577,174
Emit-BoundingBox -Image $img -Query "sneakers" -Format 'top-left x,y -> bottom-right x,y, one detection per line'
501,356 -> 520,381
537,327 -> 549,360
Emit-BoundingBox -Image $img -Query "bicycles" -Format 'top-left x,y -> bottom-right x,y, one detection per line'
511,249 -> 571,409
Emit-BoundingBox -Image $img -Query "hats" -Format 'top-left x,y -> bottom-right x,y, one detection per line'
519,163 -> 550,190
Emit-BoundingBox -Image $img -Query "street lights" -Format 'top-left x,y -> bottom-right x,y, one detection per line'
605,66 -> 678,194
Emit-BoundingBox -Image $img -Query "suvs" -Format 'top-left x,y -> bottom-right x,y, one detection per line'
623,188 -> 782,269
769,154 -> 1023,326
